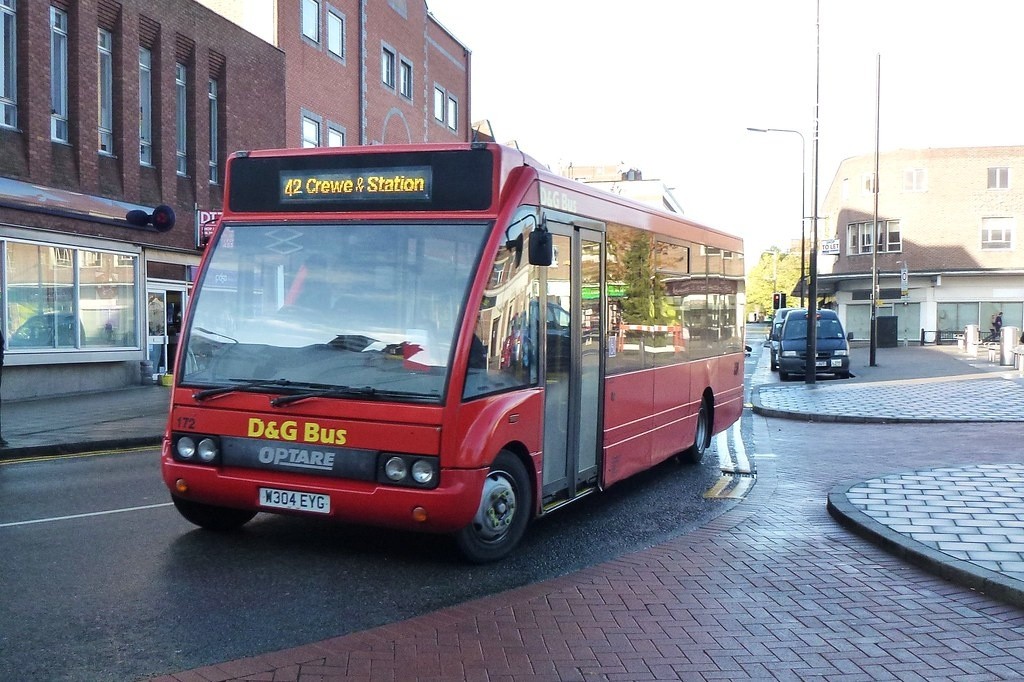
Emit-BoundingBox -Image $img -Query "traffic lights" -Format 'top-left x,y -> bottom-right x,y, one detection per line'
781,293 -> 786,308
774,294 -> 780,309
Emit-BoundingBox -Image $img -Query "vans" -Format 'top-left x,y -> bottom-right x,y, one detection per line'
779,309 -> 854,382
770,308 -> 808,371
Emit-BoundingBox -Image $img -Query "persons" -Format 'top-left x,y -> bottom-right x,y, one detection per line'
105,323 -> 113,338
986,311 -> 1003,341
174,309 -> 181,333
148,310 -> 163,374
0,330 -> 9,445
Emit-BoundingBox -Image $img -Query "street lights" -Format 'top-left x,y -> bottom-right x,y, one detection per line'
746,128 -> 804,307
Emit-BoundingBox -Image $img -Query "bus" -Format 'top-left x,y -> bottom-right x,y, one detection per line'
161,142 -> 747,564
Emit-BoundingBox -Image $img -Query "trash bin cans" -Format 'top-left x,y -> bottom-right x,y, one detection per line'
964,324 -> 979,352
999,326 -> 1019,366
